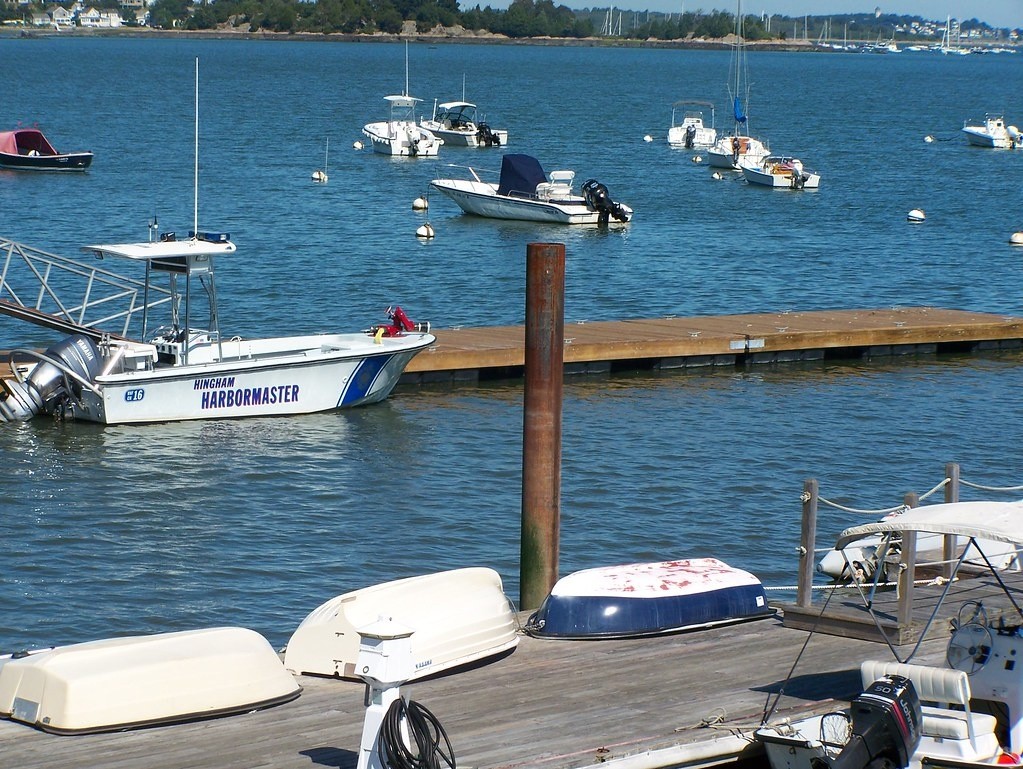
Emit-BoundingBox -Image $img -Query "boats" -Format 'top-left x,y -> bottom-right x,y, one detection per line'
737,152 -> 821,187
961,112 -> 1023,149
428,152 -> 634,226
0,499 -> 1023,768
818,36 -> 1017,56
359,37 -> 508,159
666,99 -> 717,151
0,55 -> 439,425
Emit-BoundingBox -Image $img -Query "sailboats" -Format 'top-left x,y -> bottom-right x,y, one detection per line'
707,0 -> 772,170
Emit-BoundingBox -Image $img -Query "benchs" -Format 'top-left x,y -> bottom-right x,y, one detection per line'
762,156 -> 794,170
536,170 -> 575,196
860,660 -> 1003,762
